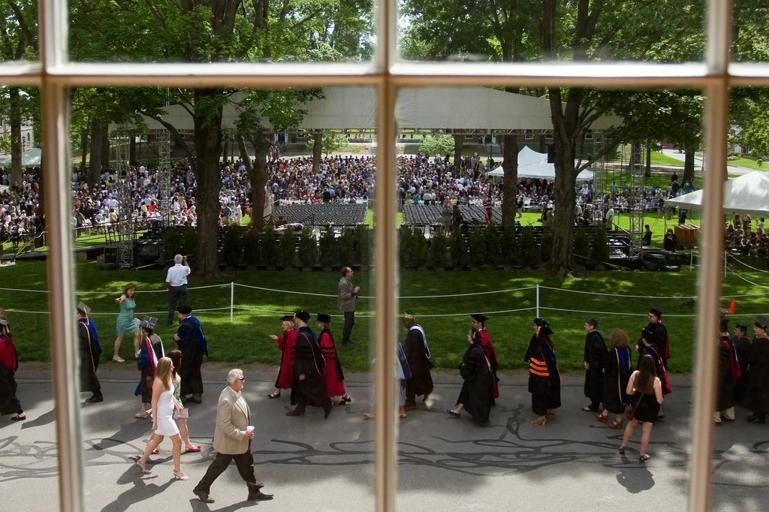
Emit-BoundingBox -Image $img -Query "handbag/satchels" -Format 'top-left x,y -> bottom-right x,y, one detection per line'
625,405 -> 635,421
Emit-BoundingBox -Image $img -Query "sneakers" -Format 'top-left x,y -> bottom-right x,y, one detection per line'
152,447 -> 160,453
187,396 -> 203,404
582,406 -> 622,428
86,393 -> 103,402
201,496 -> 207,497
11,414 -> 26,420
268,392 -> 351,419
447,409 -> 461,418
531,416 -> 546,425
184,447 -> 199,452
113,355 -> 125,362
715,414 -> 765,425
248,491 -> 276,500
641,455 -> 650,461
194,487 -> 215,503
619,446 -> 624,454
135,413 -> 148,418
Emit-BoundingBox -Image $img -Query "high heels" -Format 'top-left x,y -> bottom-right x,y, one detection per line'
134,460 -> 151,474
173,470 -> 188,480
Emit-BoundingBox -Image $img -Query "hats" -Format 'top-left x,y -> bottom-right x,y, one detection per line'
402,310 -> 417,319
139,316 -> 157,343
280,315 -> 293,321
649,308 -> 664,316
720,318 -> 768,332
296,312 -> 309,324
176,305 -> 192,315
77,300 -> 91,326
0,320 -> 10,337
317,313 -> 332,324
471,313 -> 488,321
544,326 -> 555,335
534,317 -> 549,326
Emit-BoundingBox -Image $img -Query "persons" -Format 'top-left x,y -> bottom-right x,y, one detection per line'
516,177 -> 555,221
193,369 -> 273,503
583,309 -> 672,462
0,318 -> 25,419
726,212 -> 769,259
174,304 -> 209,402
363,314 -> 433,417
527,320 -> 562,425
113,283 -> 140,362
134,316 -> 202,480
577,171 -> 694,252
166,255 -> 192,323
265,155 -> 376,231
220,161 -> 253,226
447,315 -> 499,422
267,309 -> 350,417
396,152 -> 503,223
71,153 -> 197,237
339,267 -> 360,344
715,317 -> 769,424
1,166 -> 46,239
77,302 -> 104,402
